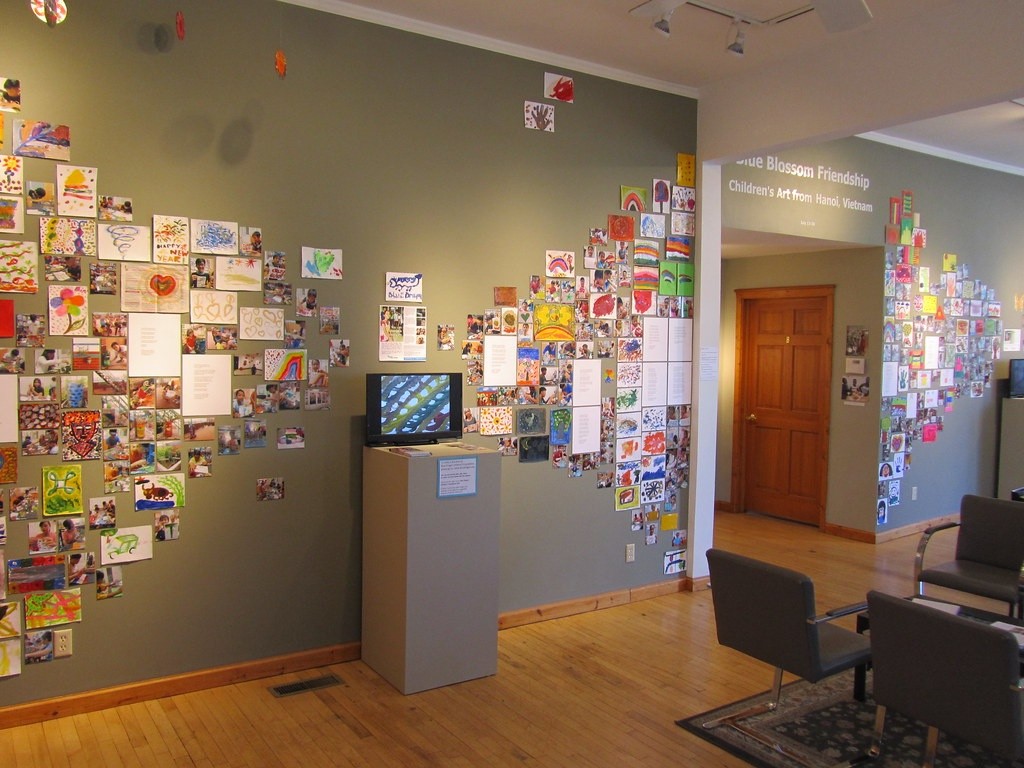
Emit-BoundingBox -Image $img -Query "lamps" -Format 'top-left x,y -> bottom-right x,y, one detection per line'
726,17 -> 746,57
653,9 -> 675,37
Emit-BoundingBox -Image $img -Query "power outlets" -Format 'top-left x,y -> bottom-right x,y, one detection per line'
627,544 -> 636,563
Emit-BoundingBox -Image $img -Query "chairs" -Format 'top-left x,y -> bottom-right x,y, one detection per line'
705,546 -> 891,768
911,494 -> 1024,625
866,589 -> 1024,768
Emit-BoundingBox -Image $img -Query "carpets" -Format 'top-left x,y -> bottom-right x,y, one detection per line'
674,660 -> 1024,768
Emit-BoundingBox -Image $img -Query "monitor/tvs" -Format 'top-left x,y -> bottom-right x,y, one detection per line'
1008,359 -> 1024,398
365,372 -> 463,446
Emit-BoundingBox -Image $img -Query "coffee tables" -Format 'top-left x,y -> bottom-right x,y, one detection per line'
852,595 -> 1024,702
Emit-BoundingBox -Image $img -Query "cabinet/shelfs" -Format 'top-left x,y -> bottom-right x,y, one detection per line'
361,444 -> 503,695
997,395 -> 1024,497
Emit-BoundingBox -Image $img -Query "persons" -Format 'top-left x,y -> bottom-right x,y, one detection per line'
291,320 -> 306,341
244,231 -> 261,257
186,329 -> 197,353
110,342 -> 128,364
92,312 -> 129,336
334,340 -> 350,367
68,555 -> 84,581
301,289 -> 318,314
308,359 -> 328,386
3,349 -> 21,364
188,258 -> 212,287
381,229 -> 694,549
266,253 -> 286,279
30,519 -> 57,549
838,245 -> 994,509
45,256 -> 81,280
251,364 -> 256,375
1,375 -> 329,664
105,428 -> 123,451
36,349 -> 69,374
